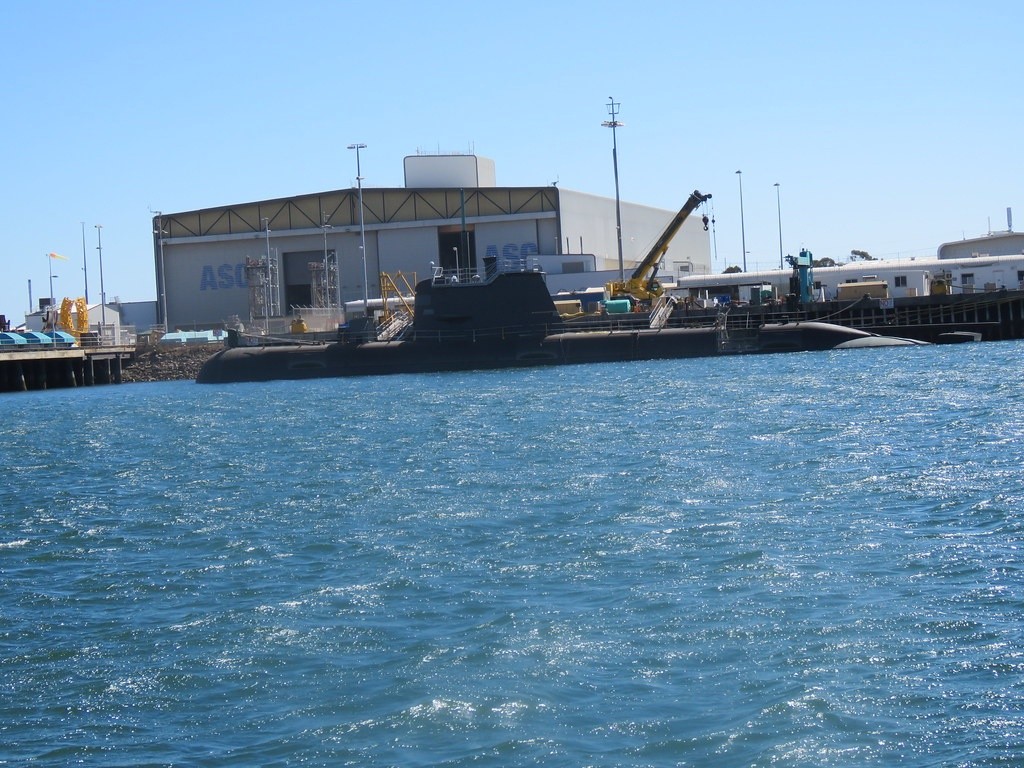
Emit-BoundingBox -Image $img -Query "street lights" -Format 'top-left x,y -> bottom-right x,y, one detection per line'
95,224 -> 106,325
735,170 -> 748,272
602,96 -> 626,281
261,217 -> 275,315
348,143 -> 368,316
150,211 -> 167,334
775,183 -> 784,269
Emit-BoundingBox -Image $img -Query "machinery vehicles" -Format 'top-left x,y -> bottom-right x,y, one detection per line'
604,190 -> 712,309
